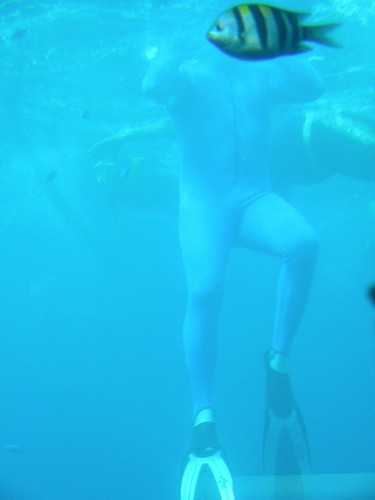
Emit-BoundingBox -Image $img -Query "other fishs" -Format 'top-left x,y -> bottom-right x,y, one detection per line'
205,3 -> 344,61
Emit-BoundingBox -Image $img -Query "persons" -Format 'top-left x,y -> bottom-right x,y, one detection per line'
143,27 -> 326,459
